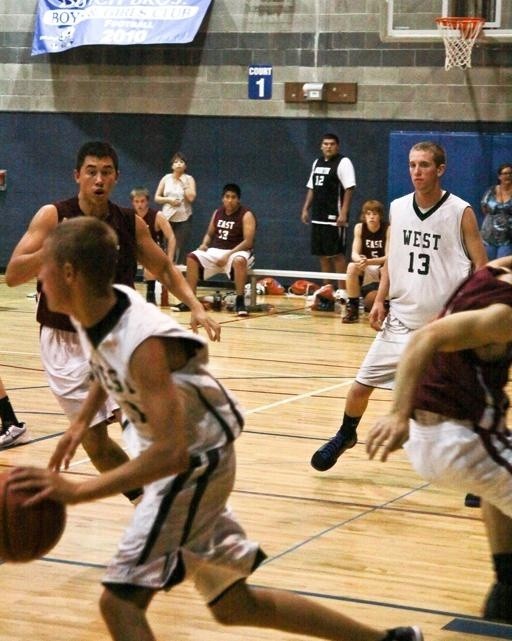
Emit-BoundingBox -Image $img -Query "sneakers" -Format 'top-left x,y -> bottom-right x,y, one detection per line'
342,305 -> 361,323
335,288 -> 347,304
312,429 -> 358,471
0,420 -> 27,448
170,301 -> 190,312
235,296 -> 248,316
395,626 -> 423,641
146,291 -> 156,304
484,583 -> 512,623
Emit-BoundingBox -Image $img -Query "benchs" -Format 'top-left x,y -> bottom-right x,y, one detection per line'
154,264 -> 347,306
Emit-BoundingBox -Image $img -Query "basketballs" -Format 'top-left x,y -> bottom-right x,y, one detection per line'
0,471 -> 67,562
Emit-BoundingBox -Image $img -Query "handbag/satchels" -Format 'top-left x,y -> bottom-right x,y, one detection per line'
287,280 -> 320,296
248,276 -> 285,295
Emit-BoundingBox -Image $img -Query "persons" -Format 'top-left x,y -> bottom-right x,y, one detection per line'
169,183 -> 257,316
153,149 -> 198,265
300,137 -> 358,303
310,136 -> 488,471
5,141 -> 222,507
129,186 -> 176,310
363,254 -> 511,624
6,215 -> 426,641
1,375 -> 26,446
342,201 -> 391,320
